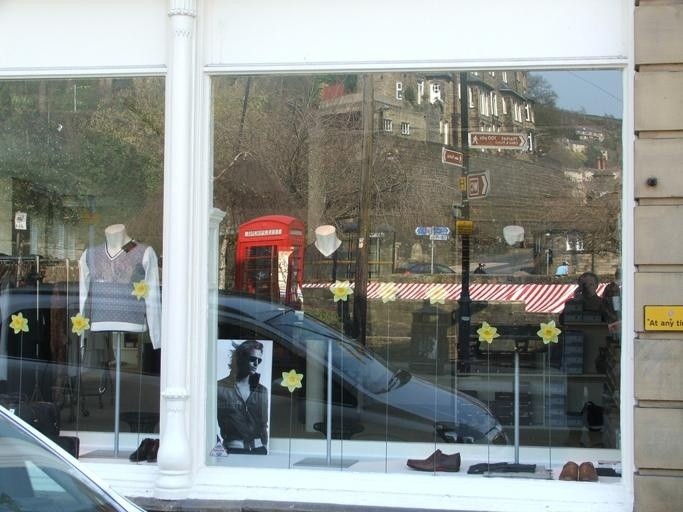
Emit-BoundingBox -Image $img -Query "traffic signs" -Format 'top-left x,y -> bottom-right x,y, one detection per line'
415,226 -> 433,236
467,131 -> 528,150
432,226 -> 450,235
440,146 -> 464,169
429,233 -> 448,241
465,171 -> 491,199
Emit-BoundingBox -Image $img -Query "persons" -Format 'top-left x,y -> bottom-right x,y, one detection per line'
551,268 -> 617,448
599,264 -> 620,414
76,222 -> 161,353
282,222 -> 370,342
214,339 -> 268,456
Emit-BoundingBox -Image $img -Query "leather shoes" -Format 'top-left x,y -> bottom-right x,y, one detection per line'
559,461 -> 579,480
578,462 -> 598,481
130,438 -> 158,463
407,450 -> 460,472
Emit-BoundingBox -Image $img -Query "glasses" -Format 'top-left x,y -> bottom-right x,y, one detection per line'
249,356 -> 262,364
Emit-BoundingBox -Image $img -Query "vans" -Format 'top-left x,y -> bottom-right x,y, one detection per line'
0,279 -> 513,448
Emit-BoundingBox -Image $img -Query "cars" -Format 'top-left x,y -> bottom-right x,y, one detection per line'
0,404 -> 149,512
394,259 -> 456,274
